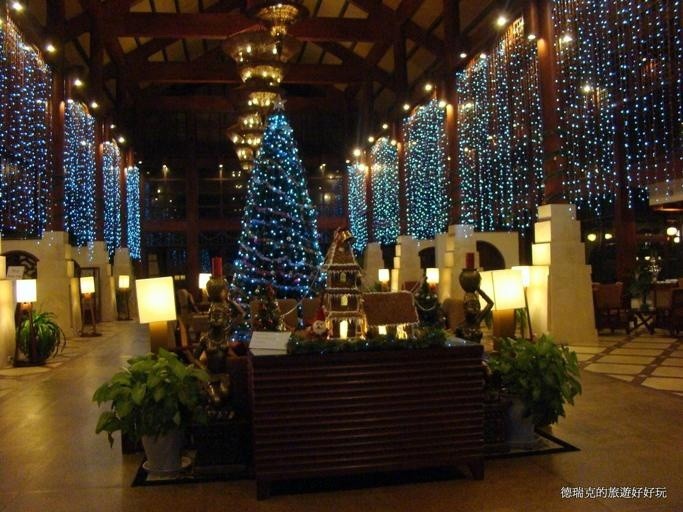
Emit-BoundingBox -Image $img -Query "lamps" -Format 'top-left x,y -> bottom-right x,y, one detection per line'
136,276 -> 178,349
77,276 -> 97,337
117,276 -> 131,321
225,3 -> 298,176
15,278 -> 37,367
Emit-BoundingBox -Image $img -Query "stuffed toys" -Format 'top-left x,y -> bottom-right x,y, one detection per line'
306,305 -> 330,344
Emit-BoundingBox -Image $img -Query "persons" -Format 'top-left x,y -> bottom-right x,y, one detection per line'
191,297 -> 242,404
455,286 -> 494,345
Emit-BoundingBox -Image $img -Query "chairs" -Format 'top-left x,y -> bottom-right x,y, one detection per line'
596,282 -> 682,337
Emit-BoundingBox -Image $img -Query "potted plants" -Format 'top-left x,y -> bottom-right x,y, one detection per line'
93,349 -> 217,478
18,312 -> 67,363
486,334 -> 582,448
